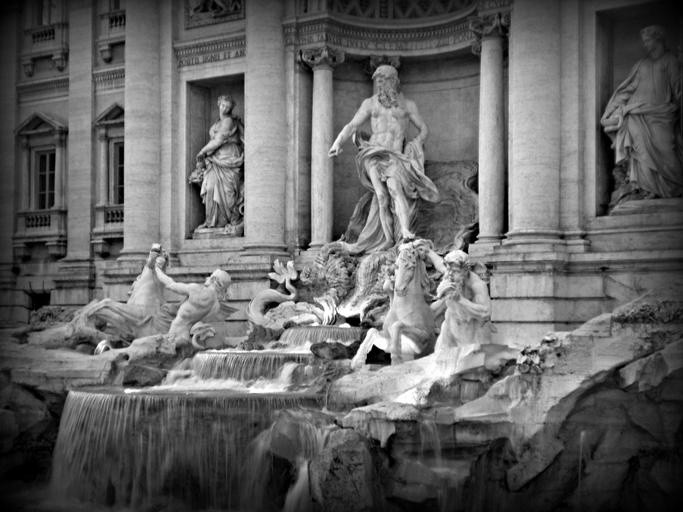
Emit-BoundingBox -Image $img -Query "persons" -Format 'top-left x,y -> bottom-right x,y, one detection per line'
601,26 -> 683,201
195,94 -> 245,228
78,242 -> 231,365
417,244 -> 492,352
327,65 -> 429,252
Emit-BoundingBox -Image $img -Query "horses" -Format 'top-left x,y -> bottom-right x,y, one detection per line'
69,243 -> 176,350
350,243 -> 437,372
